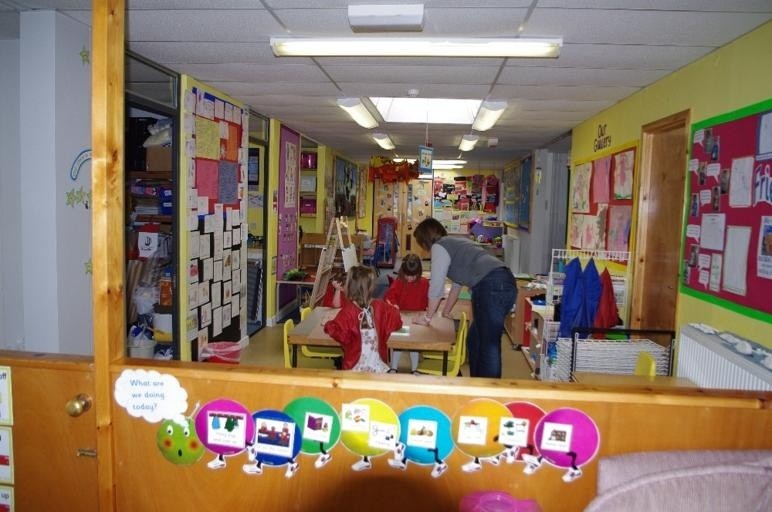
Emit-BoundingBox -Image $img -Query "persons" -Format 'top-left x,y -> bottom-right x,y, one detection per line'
323,264 -> 402,374
321,271 -> 350,368
383,252 -> 430,375
411,217 -> 520,379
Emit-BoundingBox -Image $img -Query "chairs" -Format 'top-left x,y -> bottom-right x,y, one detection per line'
413,311 -> 469,378
282,304 -> 344,369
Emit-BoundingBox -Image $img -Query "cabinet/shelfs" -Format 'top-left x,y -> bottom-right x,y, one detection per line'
503,273 -> 546,379
127,173 -> 176,222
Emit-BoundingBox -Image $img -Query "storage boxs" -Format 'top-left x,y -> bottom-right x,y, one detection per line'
145,144 -> 172,171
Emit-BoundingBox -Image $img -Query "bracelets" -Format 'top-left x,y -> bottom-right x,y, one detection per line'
423,315 -> 431,323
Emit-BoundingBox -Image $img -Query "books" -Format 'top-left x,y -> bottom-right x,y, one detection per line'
135,203 -> 161,215
390,325 -> 410,337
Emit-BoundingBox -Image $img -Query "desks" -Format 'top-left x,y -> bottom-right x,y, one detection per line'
289,306 -> 457,374
274,278 -> 313,326
